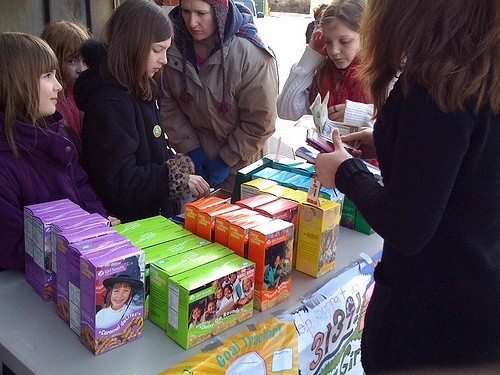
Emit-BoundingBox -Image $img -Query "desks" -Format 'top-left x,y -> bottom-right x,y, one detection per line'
0,224 -> 385,375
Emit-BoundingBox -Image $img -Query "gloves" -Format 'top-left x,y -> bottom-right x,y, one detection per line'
205,156 -> 230,186
187,148 -> 207,176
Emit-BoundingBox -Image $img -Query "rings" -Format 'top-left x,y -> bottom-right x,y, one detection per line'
334,106 -> 338,112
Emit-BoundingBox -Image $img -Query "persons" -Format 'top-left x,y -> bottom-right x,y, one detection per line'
189,271 -> 254,330
96,263 -> 144,329
264,255 -> 283,288
160,0 -> 279,193
0,32 -> 121,271
41,20 -> 90,140
74,0 -> 211,224
314,0 -> 500,375
306,4 -> 329,44
276,0 -> 368,122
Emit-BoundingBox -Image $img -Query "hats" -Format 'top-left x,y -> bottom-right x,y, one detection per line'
103,256 -> 144,297
179,0 -> 231,113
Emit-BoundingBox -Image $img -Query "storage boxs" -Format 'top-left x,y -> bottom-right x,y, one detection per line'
23,198 -> 146,357
184,195 -> 295,313
110,215 -> 256,351
232,154 -> 376,279
